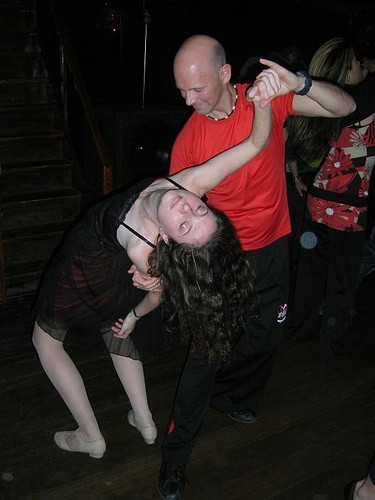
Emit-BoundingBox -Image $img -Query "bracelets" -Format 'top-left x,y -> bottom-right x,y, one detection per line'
294,176 -> 301,182
294,71 -> 312,96
133,308 -> 141,319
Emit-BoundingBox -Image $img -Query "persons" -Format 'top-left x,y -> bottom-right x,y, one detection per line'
128,35 -> 357,500
282,38 -> 375,380
31,71 -> 282,458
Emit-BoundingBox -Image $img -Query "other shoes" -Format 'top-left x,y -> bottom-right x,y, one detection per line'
343,480 -> 358,500
157,461 -> 191,500
126,408 -> 158,445
225,408 -> 258,424
53,426 -> 107,459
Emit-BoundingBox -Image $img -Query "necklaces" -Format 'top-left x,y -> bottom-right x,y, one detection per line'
206,85 -> 238,121
